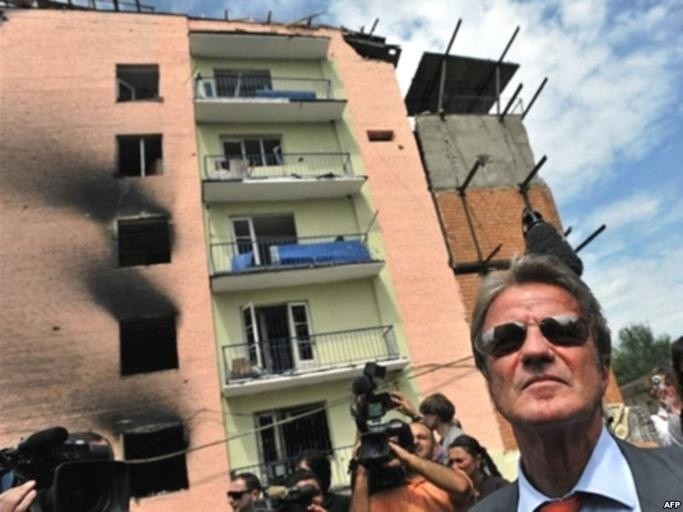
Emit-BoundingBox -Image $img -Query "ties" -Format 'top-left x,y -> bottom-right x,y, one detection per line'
538,496 -> 580,512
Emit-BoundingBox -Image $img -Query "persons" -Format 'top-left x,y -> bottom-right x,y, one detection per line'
226,389 -> 510,511
0,477 -> 40,512
644,366 -> 680,447
667,336 -> 682,450
465,249 -> 681,511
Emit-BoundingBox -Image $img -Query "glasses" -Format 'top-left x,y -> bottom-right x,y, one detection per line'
227,492 -> 247,499
474,314 -> 592,357
